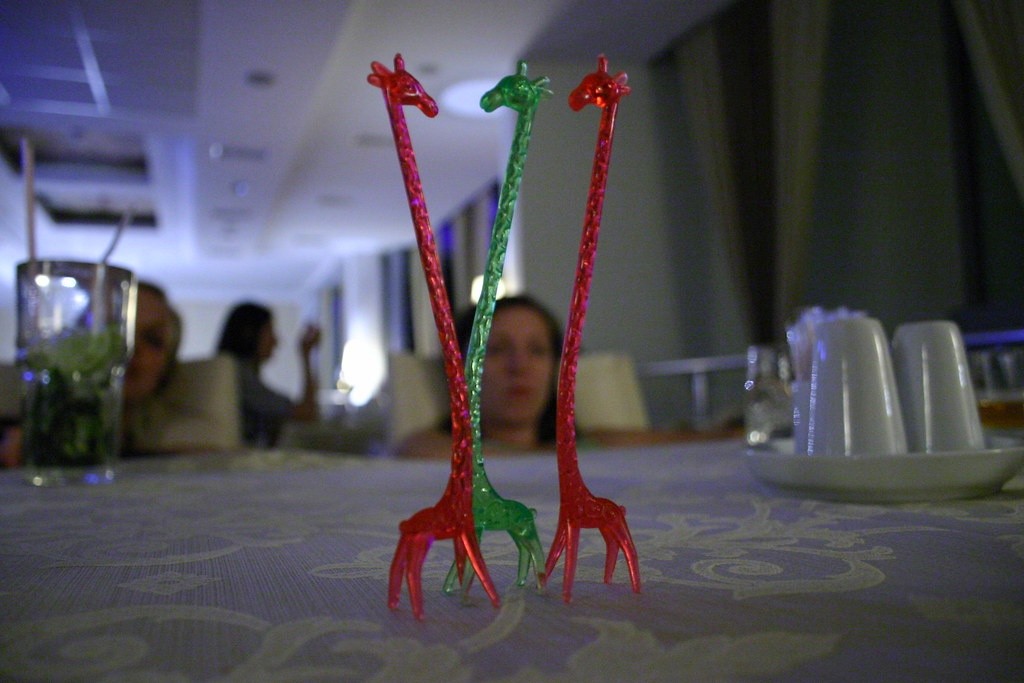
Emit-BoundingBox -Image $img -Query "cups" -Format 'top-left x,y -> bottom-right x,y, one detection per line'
807,319 -> 908,458
742,349 -> 794,435
16,261 -> 137,488
793,383 -> 810,459
893,322 -> 985,455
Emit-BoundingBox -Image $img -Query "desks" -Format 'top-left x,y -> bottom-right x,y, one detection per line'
0,439 -> 1024,683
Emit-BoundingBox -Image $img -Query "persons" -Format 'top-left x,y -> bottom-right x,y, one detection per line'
217,300 -> 323,451
0,281 -> 183,474
394,294 -> 580,459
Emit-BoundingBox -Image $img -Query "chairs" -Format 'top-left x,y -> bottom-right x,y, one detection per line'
387,352 -> 650,438
131,357 -> 239,453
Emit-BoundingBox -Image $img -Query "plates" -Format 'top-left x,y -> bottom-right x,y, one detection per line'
743,438 -> 1024,500
